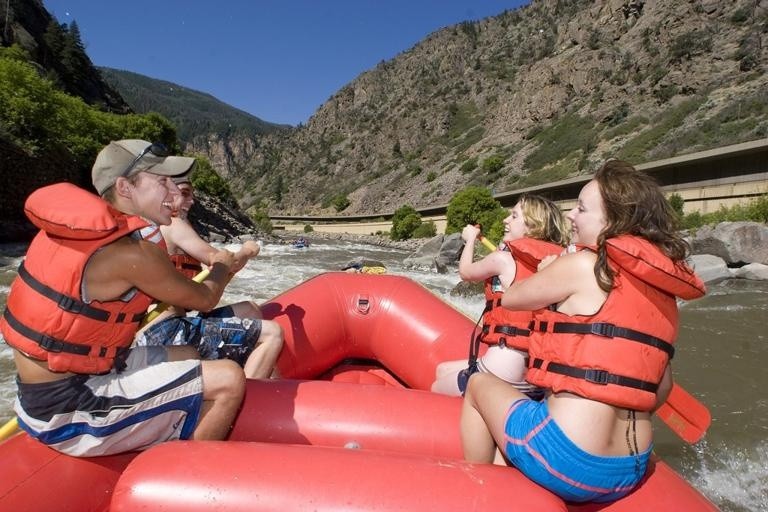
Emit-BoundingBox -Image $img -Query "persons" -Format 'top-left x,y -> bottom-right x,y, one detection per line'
432,192 -> 570,395
132,174 -> 285,379
1,139 -> 246,461
461,162 -> 707,504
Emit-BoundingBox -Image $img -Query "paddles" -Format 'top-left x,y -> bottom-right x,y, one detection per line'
473,224 -> 711,444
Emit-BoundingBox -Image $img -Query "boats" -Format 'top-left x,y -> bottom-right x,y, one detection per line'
1,273 -> 724,512
293,237 -> 309,249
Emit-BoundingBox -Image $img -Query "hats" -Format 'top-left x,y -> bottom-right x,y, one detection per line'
92,139 -> 197,196
